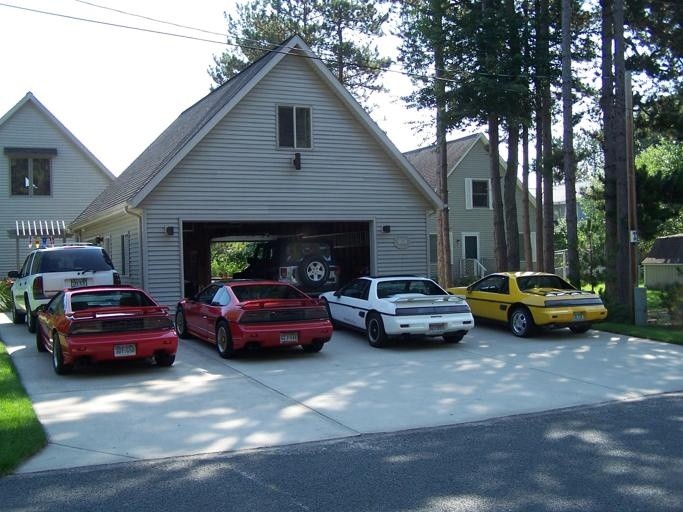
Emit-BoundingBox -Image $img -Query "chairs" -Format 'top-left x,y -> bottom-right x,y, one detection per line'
119,297 -> 137,307
71,302 -> 87,311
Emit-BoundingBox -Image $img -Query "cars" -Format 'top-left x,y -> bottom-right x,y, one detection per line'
319,273 -> 476,346
37,283 -> 179,373
175,278 -> 334,358
445,270 -> 608,338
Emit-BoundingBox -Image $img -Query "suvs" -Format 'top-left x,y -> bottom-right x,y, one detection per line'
9,244 -> 122,331
271,238 -> 342,293
